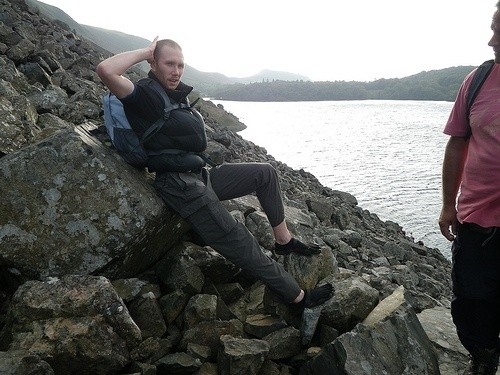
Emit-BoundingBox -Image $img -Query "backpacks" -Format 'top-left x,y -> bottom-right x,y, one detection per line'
101,77 -> 190,168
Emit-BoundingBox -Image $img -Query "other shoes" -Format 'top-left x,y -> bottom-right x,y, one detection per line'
274,238 -> 323,257
292,283 -> 335,314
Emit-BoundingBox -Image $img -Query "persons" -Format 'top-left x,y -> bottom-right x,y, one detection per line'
439,0 -> 500,375
96,36 -> 335,317
